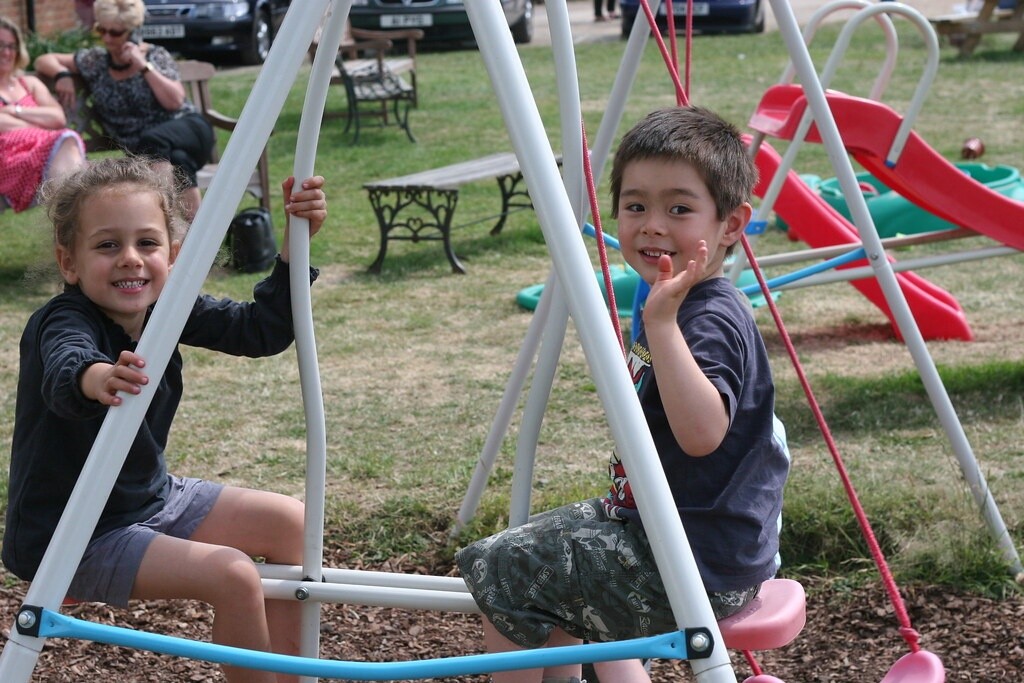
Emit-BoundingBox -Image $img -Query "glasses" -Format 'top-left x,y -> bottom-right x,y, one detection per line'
95,22 -> 127,38
0,41 -> 18,52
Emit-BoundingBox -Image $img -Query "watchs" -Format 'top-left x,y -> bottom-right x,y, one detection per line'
140,62 -> 152,75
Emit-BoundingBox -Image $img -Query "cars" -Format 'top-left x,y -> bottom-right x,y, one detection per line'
620,1 -> 766,37
135,1 -> 292,64
349,1 -> 533,57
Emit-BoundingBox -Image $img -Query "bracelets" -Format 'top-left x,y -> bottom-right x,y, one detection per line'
53,71 -> 74,84
15,105 -> 22,118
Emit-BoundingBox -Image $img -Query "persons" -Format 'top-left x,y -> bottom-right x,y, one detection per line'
454,106 -> 790,683
0,162 -> 328,683
0,0 -> 218,225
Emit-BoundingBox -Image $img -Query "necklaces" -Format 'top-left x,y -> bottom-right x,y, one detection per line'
106,50 -> 133,71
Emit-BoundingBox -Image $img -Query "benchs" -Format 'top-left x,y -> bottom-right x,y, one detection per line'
0,62 -> 275,215
334,53 -> 417,145
363,149 -> 592,276
928,9 -> 1013,23
305,9 -> 425,121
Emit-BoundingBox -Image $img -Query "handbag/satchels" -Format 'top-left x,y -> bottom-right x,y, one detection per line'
223,207 -> 277,273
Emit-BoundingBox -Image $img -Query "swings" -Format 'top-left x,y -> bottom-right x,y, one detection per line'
578,2 -> 948,683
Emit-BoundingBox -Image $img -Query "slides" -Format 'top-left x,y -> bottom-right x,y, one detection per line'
738,133 -> 975,345
747,84 -> 1024,252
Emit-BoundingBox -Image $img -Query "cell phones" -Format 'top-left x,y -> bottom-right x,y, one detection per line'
129,29 -> 139,45
